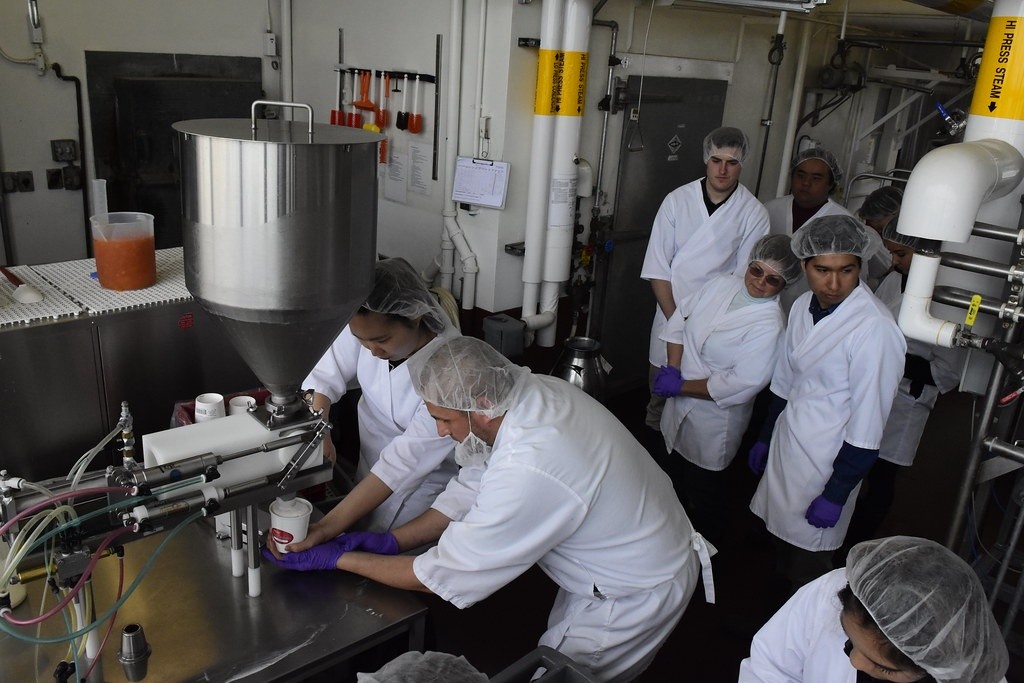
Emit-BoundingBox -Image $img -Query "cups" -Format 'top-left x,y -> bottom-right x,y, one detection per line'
229,396 -> 257,416
194,392 -> 227,424
269,497 -> 313,554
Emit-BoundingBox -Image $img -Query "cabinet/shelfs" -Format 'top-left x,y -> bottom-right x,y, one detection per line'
0,247 -> 265,479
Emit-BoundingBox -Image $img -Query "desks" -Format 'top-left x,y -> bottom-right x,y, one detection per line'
0,496 -> 429,683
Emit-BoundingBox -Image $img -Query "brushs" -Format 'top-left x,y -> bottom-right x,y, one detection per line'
396,74 -> 409,130
330,69 -> 344,126
407,75 -> 422,133
380,73 -> 390,163
348,70 -> 360,128
353,70 -> 375,111
362,69 -> 384,134
375,72 -> 386,128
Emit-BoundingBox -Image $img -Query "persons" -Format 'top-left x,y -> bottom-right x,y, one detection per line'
654,231 -> 803,545
718,202 -> 907,639
259,336 -> 718,683
267,258 -> 465,561
836,216 -> 965,556
860,186 -> 903,290
639,126 -> 770,432
763,148 -> 853,311
738,535 -> 1009,682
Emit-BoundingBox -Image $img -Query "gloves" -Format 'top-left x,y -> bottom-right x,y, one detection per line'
263,542 -> 344,570
334,531 -> 399,556
651,365 -> 685,398
806,495 -> 842,528
748,441 -> 768,475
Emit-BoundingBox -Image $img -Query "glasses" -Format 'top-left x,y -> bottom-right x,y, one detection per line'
750,263 -> 780,287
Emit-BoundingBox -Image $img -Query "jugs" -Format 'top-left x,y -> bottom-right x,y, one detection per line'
88,211 -> 157,291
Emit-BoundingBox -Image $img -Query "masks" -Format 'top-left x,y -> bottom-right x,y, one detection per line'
454,433 -> 490,469
